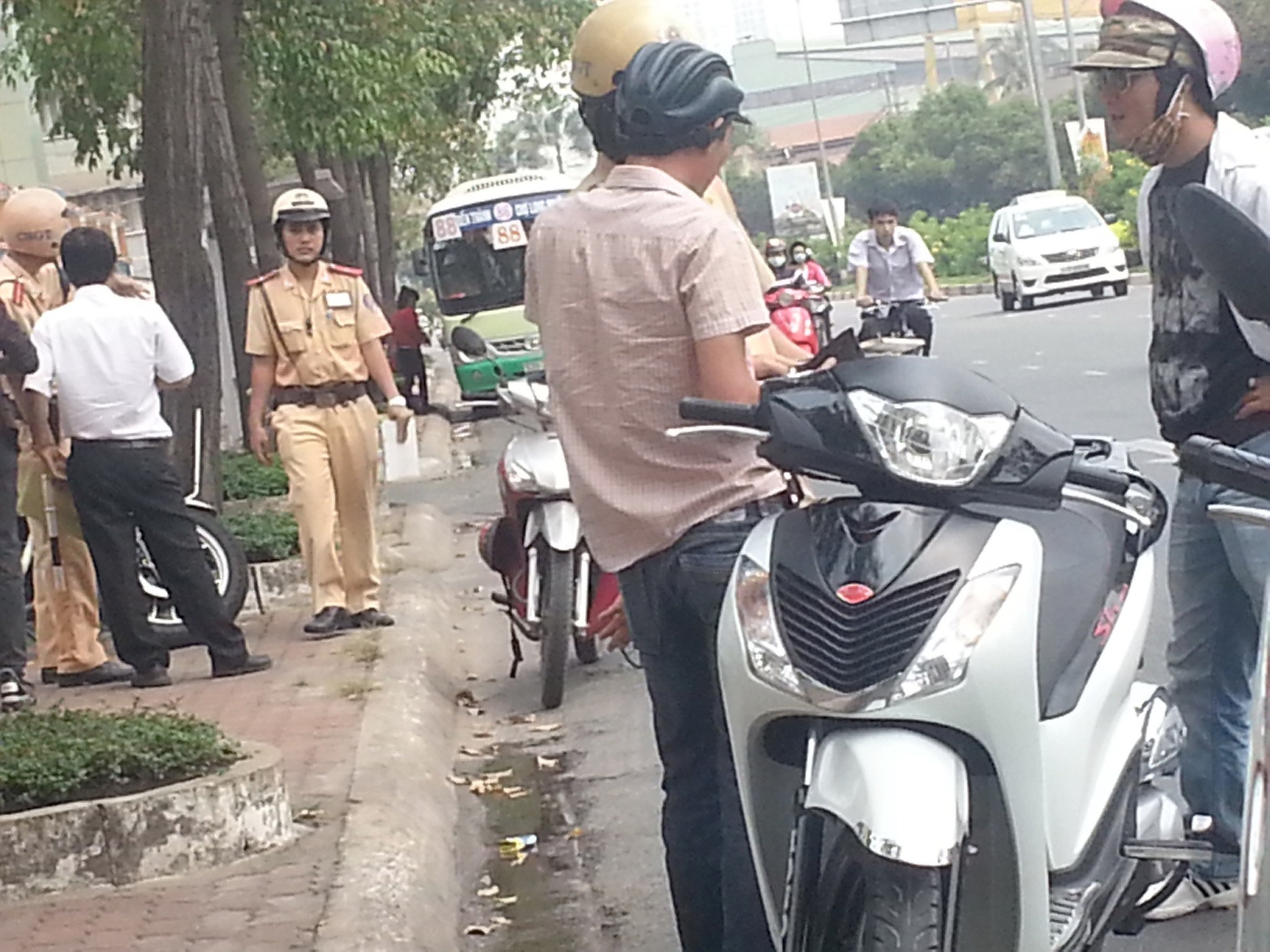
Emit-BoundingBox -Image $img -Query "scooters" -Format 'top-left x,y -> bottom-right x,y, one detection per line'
803,283 -> 831,346
663,353 -> 1270,951
768,288 -> 821,354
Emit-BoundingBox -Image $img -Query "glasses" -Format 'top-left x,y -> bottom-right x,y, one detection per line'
1086,67 -> 1154,96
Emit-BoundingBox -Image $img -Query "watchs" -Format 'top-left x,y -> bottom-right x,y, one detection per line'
389,396 -> 407,407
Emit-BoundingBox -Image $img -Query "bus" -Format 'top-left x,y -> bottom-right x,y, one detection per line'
411,165 -> 580,399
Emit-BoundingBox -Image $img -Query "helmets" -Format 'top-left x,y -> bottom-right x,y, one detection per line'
270,187 -> 331,233
611,38 -> 754,156
765,237 -> 787,256
478,516 -> 508,573
0,188 -> 72,257
567,0 -> 671,98
1097,1 -> 1244,101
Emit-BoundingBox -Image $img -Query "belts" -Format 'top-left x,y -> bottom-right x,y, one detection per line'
279,382 -> 365,405
71,438 -> 160,449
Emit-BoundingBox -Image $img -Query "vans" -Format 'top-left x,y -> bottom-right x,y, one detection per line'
987,187 -> 1129,311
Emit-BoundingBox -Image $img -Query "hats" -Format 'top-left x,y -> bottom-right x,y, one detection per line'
1067,7 -> 1202,71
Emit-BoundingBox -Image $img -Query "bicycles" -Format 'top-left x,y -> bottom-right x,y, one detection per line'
867,295 -> 951,339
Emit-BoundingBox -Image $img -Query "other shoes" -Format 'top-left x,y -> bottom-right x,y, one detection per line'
41,667 -> 58,683
129,662 -> 172,687
212,653 -> 271,677
1130,862 -> 1240,919
351,609 -> 394,626
58,658 -> 136,687
0,678 -> 30,703
304,605 -> 354,633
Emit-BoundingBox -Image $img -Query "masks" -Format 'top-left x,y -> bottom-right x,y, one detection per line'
1123,74 -> 1194,168
767,256 -> 786,269
793,253 -> 806,264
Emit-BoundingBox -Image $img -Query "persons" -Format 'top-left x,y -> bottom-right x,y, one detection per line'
389,284 -> 437,411
1071,0 -> 1270,922
571,0 -> 947,386
523,41 -> 782,952
0,187 -> 273,710
245,189 -> 415,633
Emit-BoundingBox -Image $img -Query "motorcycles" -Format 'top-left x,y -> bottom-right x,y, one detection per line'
446,324 -> 621,710
136,410 -> 250,649
